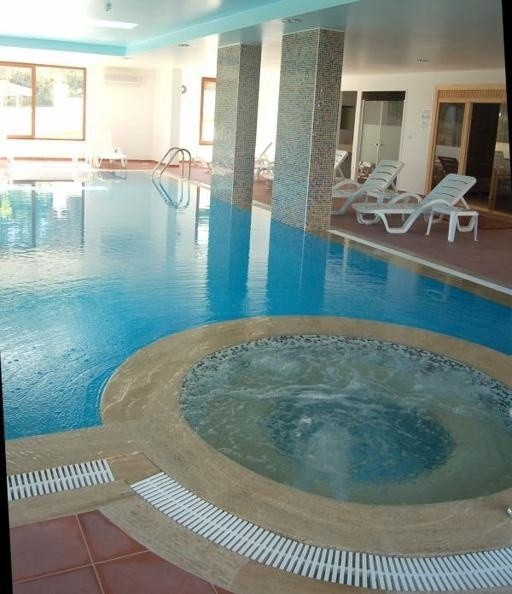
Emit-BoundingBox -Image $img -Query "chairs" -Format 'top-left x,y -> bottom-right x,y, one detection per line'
331,158 -> 405,215
350,172 -> 478,232
206,140 -> 348,188
86,127 -> 128,169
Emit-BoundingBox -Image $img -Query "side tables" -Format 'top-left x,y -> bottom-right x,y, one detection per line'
424,204 -> 480,240
364,188 -> 408,222
